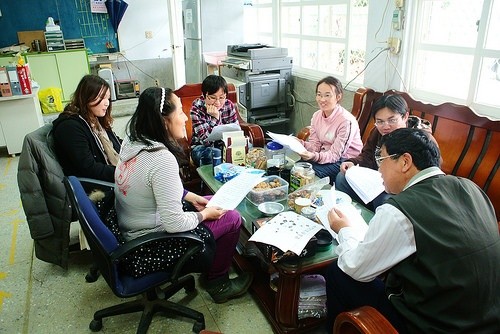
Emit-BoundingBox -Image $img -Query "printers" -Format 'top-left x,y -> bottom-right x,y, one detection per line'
220,44 -> 294,121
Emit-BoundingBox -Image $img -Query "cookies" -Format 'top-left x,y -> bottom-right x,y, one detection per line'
249,177 -> 284,203
245,148 -> 267,169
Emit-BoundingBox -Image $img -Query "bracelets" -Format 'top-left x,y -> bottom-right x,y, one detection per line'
182,190 -> 188,200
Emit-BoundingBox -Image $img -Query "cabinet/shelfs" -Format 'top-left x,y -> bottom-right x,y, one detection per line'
26,49 -> 92,104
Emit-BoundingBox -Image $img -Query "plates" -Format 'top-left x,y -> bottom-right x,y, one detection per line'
312,190 -> 352,208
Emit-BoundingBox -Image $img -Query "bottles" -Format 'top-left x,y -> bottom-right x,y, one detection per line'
35,39 -> 41,51
265,141 -> 285,175
290,162 -> 315,193
211,139 -> 225,176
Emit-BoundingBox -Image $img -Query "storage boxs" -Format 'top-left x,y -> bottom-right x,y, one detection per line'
204,52 -> 226,64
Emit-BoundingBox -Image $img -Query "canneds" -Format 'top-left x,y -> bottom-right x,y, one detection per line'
293,198 -> 316,222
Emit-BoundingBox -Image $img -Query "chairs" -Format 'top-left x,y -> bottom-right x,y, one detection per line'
333,305 -> 398,334
17,82 -> 266,334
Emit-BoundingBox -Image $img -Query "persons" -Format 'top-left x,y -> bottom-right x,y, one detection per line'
335,93 -> 439,213
324,128 -> 500,334
113,87 -> 254,305
49,75 -> 124,203
293,76 -> 363,179
189,74 -> 244,166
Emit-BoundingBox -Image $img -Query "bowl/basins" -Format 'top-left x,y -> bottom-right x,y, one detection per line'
313,229 -> 333,246
246,175 -> 289,206
258,202 -> 285,216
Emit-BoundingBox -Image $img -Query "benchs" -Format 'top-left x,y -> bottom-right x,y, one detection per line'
297,88 -> 500,228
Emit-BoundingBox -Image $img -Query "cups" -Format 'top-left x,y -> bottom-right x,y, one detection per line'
294,198 -> 316,221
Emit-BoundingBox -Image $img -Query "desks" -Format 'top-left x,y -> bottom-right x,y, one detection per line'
0,86 -> 44,158
208,63 -> 225,77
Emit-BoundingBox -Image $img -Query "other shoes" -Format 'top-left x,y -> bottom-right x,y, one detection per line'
199,266 -> 254,304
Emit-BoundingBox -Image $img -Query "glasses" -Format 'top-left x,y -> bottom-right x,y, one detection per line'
206,94 -> 226,101
315,92 -> 336,100
375,154 -> 403,168
374,115 -> 406,127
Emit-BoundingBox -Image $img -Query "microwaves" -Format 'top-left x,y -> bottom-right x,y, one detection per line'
115,79 -> 140,99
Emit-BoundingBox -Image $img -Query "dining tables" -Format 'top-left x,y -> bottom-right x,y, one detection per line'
199,155 -> 377,334
